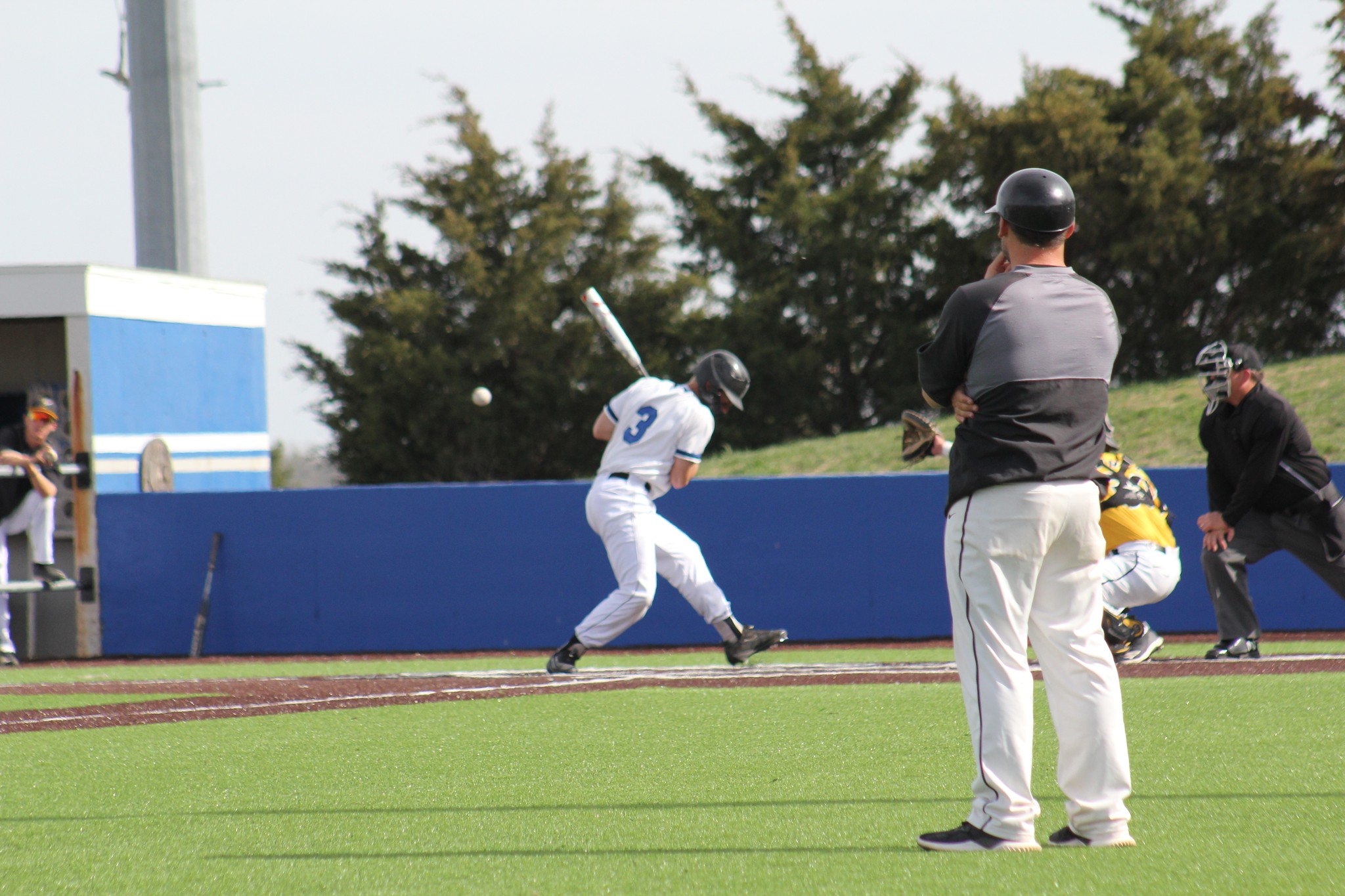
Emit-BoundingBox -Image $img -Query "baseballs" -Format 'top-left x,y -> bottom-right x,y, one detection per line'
472,386 -> 492,407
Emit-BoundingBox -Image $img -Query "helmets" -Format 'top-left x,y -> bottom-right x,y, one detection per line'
985,168 -> 1076,232
692,353 -> 750,411
1199,343 -> 1261,372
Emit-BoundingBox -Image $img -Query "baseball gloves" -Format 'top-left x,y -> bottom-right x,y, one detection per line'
892,409 -> 946,473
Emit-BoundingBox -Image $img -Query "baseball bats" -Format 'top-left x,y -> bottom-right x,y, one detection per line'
579,285 -> 650,378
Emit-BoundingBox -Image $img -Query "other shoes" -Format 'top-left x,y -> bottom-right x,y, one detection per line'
0,651 -> 19,667
32,563 -> 67,581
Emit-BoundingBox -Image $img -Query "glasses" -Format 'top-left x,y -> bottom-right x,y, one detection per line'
27,411 -> 55,425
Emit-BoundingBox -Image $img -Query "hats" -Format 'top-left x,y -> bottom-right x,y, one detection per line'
26,394 -> 59,420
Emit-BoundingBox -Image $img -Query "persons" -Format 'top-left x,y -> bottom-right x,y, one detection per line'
909,168 -> 1137,852
545,349 -> 788,671
917,409 -> 1183,666
0,396 -> 68,665
1195,343 -> 1345,660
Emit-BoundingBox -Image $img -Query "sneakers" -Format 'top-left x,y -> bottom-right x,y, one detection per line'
547,645 -> 578,674
917,821 -> 1042,853
1205,637 -> 1260,659
1047,825 -> 1136,847
1117,621 -> 1164,664
719,625 -> 787,666
